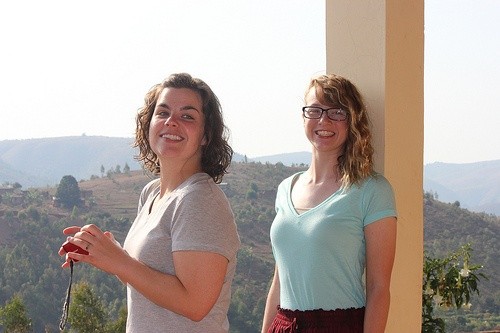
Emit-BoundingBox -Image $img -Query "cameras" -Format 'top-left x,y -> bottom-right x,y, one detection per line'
62,232 -> 94,263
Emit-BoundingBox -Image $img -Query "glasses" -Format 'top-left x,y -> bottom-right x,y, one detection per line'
302,106 -> 348,121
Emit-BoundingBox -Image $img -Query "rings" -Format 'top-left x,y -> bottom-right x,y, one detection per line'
85,244 -> 90,251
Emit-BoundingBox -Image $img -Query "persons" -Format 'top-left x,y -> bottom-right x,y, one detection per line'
261,74 -> 397,333
58,72 -> 239,332
